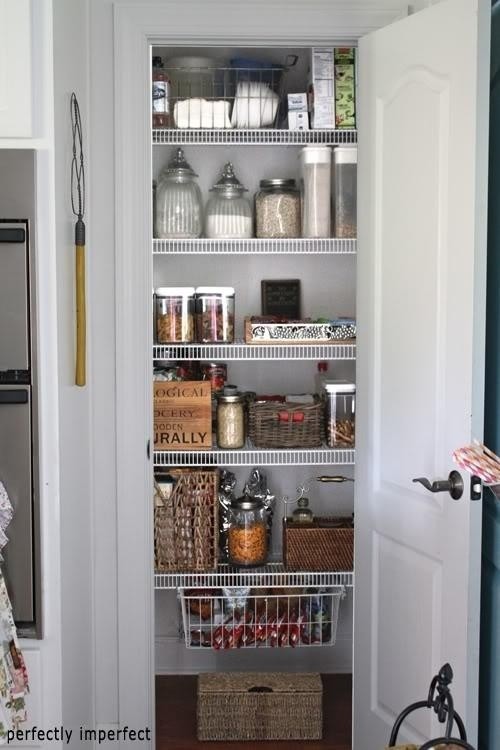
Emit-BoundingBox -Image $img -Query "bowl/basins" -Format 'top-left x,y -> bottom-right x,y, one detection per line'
172,80 -> 280,133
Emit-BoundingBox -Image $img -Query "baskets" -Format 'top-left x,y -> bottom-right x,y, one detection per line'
281,476 -> 354,571
153,466 -> 220,571
195,673 -> 323,740
248,401 -> 324,448
174,587 -> 347,650
152,53 -> 299,129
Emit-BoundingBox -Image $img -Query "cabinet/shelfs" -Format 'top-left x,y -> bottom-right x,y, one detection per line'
105,0 -> 383,674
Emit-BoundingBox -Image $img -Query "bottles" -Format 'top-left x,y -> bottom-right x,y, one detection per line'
227,492 -> 315,568
218,394 -> 246,449
154,144 -> 358,241
152,55 -> 171,131
155,287 -> 236,344
320,376 -> 355,448
